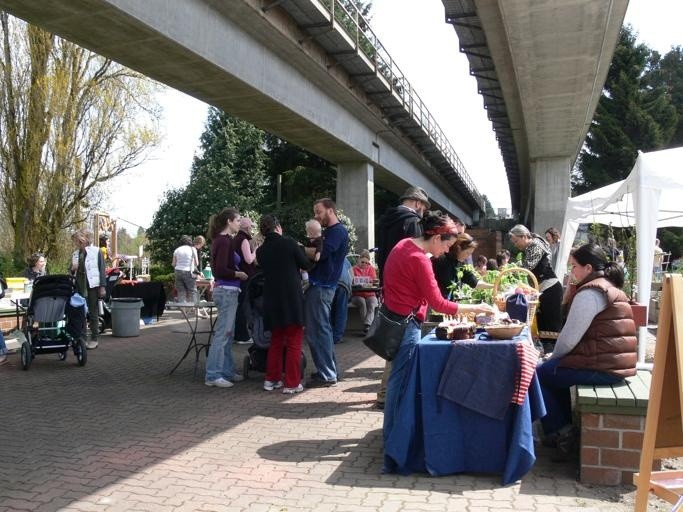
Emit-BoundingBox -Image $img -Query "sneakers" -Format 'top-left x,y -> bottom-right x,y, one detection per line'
282,384 -> 304,394
238,338 -> 255,344
363,325 -> 372,332
205,377 -> 235,388
225,373 -> 245,381
0,354 -> 9,366
263,380 -> 284,390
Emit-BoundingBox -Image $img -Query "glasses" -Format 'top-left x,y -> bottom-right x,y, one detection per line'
361,260 -> 370,265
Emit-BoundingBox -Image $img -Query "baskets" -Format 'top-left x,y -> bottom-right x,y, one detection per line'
493,267 -> 540,326
486,326 -> 521,339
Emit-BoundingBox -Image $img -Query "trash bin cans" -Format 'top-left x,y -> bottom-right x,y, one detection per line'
110,297 -> 144,336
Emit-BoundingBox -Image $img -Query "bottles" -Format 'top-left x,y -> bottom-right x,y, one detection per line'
24,280 -> 33,297
355,276 -> 379,287
204,263 -> 212,280
447,312 -> 476,338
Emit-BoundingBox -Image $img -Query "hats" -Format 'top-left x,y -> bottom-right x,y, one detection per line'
399,185 -> 431,208
240,218 -> 257,229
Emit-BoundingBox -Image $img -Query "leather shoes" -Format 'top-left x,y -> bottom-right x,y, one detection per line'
540,432 -> 559,448
548,426 -> 579,463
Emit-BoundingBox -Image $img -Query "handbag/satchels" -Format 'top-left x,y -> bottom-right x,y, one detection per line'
191,263 -> 200,279
362,311 -> 407,362
87,341 -> 100,349
305,378 -> 338,389
311,371 -> 322,378
194,310 -> 205,318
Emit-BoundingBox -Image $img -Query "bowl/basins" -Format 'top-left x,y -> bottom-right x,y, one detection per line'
485,323 -> 525,339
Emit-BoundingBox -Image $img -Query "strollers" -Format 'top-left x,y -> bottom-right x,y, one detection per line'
11,273 -> 90,368
91,266 -> 130,335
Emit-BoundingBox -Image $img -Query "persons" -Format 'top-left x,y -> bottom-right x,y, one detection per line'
171,232 -> 200,304
191,234 -> 206,280
203,197 -> 348,394
330,249 -> 379,343
0,272 -> 11,366
68,231 -> 107,352
99,235 -> 113,264
652,238 -> 665,281
27,251 -> 50,281
508,224 -> 638,461
374,187 -> 510,409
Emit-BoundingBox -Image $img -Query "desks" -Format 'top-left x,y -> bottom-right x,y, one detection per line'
165,302 -> 221,380
180,282 -> 215,319
112,282 -> 163,322
415,322 -> 530,478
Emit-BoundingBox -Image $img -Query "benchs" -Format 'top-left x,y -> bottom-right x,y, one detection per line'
577,370 -> 653,488
